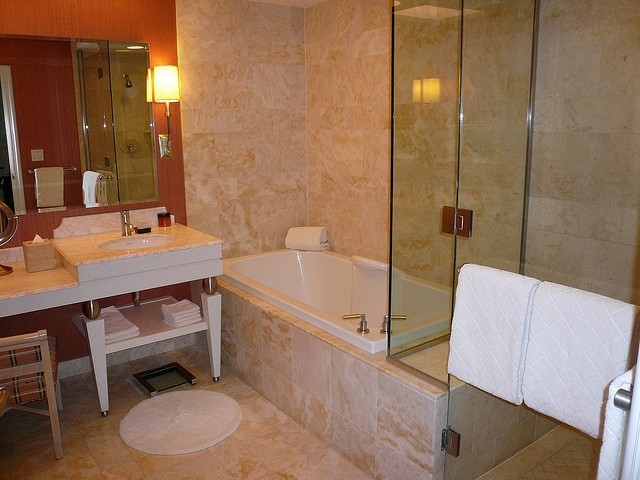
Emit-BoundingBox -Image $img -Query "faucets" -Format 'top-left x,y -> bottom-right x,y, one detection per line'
120,209 -> 134,237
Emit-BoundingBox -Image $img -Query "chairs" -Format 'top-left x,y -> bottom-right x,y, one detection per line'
0,329 -> 64,460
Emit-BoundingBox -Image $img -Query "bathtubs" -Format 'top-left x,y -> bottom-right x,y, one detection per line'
216,247 -> 457,354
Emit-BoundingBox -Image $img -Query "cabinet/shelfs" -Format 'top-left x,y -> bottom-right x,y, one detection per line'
70,291 -> 222,417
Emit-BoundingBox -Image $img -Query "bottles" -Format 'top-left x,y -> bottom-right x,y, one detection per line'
157,212 -> 171,227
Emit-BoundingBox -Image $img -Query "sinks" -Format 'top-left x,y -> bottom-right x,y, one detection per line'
51,222 -> 232,284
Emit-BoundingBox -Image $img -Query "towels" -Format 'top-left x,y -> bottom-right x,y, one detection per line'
33,166 -> 64,207
284,225 -> 331,252
446,264 -> 540,406
96,305 -> 140,344
82,170 -> 107,207
160,297 -> 202,328
596,363 -> 636,480
520,282 -> 640,442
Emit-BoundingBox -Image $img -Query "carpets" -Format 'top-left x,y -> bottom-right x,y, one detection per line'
118,390 -> 243,456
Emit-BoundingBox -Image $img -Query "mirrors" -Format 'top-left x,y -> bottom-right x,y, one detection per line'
0,200 -> 18,277
0,32 -> 159,218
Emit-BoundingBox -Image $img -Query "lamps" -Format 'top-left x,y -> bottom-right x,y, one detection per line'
154,66 -> 181,159
